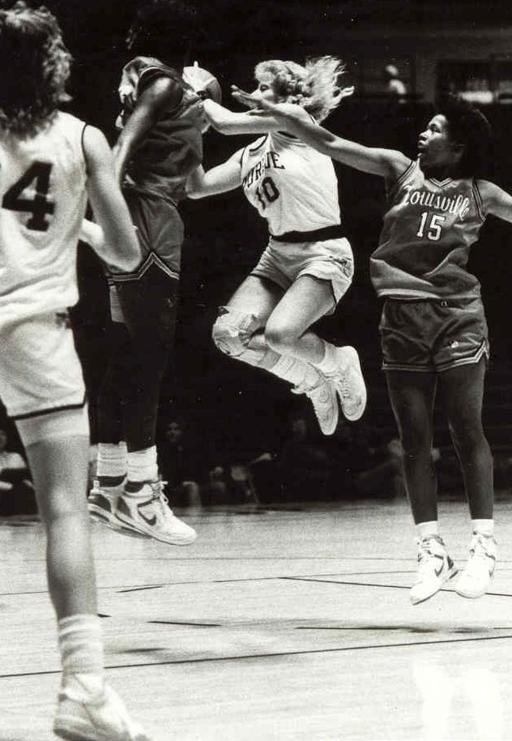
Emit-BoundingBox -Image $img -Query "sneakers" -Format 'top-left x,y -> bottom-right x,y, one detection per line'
410,536 -> 457,605
112,482 -> 196,545
457,530 -> 496,598
53,687 -> 153,740
87,476 -> 152,540
291,369 -> 338,436
325,345 -> 367,422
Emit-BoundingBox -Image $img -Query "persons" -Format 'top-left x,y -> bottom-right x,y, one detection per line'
230,82 -> 511,604
1,0 -> 142,741
380,63 -> 409,102
3,416 -> 512,516
87,0 -> 205,547
184,53 -> 367,438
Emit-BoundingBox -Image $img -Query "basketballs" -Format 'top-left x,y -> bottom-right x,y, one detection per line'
182,66 -> 222,132
